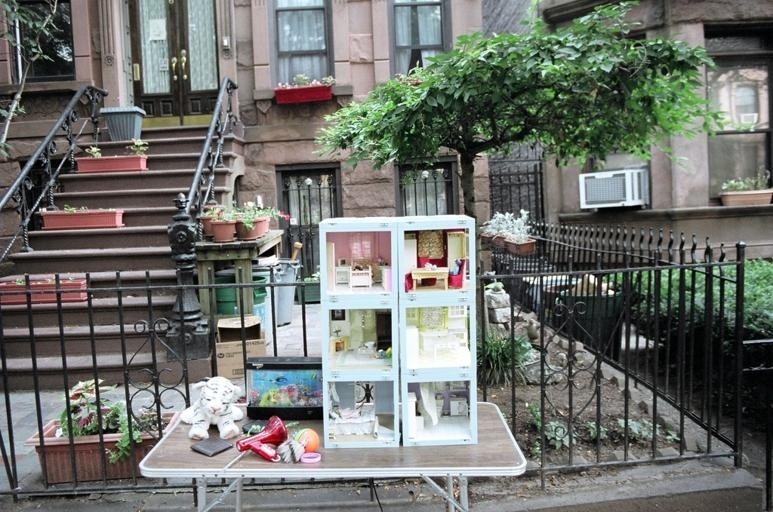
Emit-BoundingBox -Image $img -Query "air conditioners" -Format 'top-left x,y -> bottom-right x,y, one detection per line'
578,168 -> 650,212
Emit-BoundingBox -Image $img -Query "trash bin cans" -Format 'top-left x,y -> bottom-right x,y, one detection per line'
544,284 -> 624,361
273,258 -> 300,327
208,275 -> 267,339
215,266 -> 273,345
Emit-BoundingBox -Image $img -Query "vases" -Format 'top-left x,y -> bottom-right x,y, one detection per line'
24,410 -> 180,485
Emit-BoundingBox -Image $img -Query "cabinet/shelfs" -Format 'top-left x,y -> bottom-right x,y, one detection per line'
319,212 -> 481,451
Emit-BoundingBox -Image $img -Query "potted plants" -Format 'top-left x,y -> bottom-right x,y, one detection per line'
0,278 -> 87,304
74,137 -> 149,172
273,73 -> 335,105
480,209 -> 535,256
196,194 -> 294,242
39,204 -> 125,231
716,166 -> 773,207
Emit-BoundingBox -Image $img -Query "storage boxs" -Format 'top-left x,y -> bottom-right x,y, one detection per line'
213,313 -> 266,381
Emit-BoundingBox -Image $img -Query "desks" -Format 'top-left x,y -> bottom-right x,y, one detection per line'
194,229 -> 284,333
136,399 -> 528,512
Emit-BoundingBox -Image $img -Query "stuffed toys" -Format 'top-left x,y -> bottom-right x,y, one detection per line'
176,375 -> 246,442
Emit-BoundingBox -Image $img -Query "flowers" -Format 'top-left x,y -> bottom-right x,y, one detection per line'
54,378 -> 168,465
